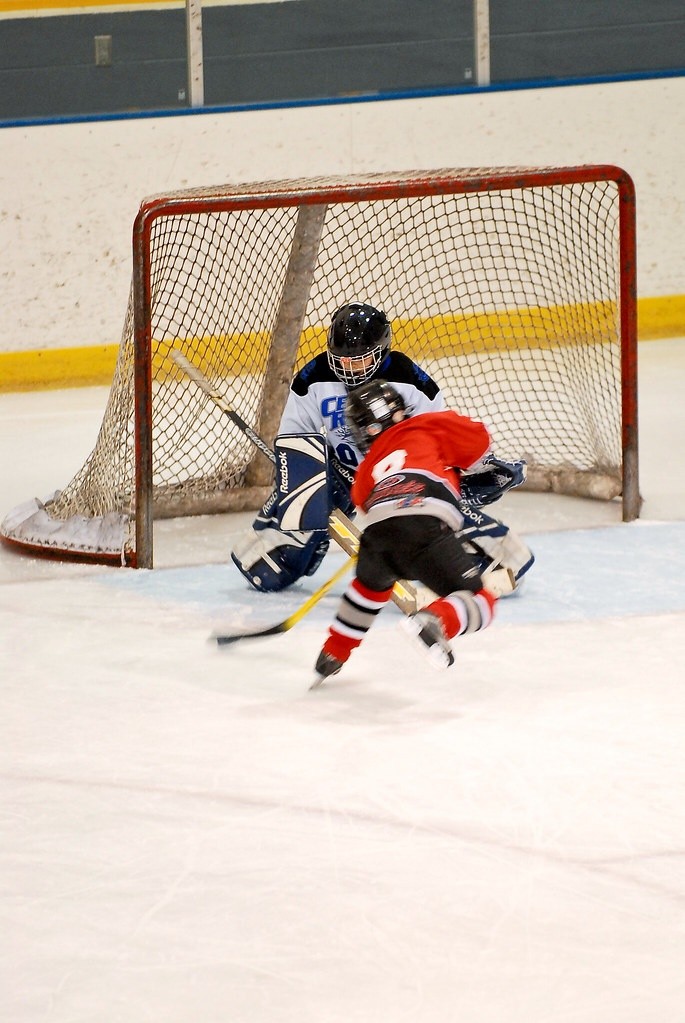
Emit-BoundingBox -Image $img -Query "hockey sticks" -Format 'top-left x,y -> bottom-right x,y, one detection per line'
165,344 -> 522,621
211,554 -> 358,648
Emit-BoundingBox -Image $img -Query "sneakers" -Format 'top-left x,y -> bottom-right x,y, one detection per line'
409,608 -> 453,668
308,651 -> 343,692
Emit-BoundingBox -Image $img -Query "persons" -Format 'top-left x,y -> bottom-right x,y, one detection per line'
231,300 -> 535,692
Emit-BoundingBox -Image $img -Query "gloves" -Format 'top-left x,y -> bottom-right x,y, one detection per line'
461,473 -> 503,511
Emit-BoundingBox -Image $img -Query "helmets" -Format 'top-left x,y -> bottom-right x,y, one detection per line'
344,381 -> 411,453
326,302 -> 391,387
